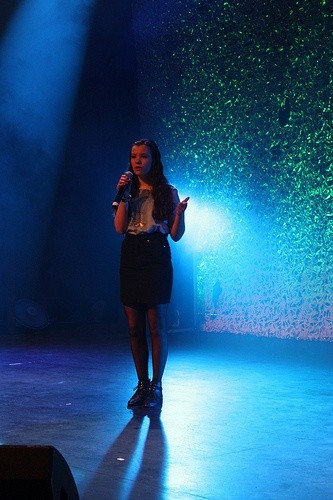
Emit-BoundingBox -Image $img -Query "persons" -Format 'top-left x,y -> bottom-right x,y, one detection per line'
113,139 -> 190,407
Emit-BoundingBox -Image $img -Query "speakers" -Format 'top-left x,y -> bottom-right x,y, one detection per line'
0,444 -> 81,500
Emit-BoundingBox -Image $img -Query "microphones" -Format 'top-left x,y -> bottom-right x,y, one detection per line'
112,172 -> 133,212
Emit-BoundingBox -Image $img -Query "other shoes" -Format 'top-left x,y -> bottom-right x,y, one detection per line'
144,381 -> 162,408
128,378 -> 152,407
